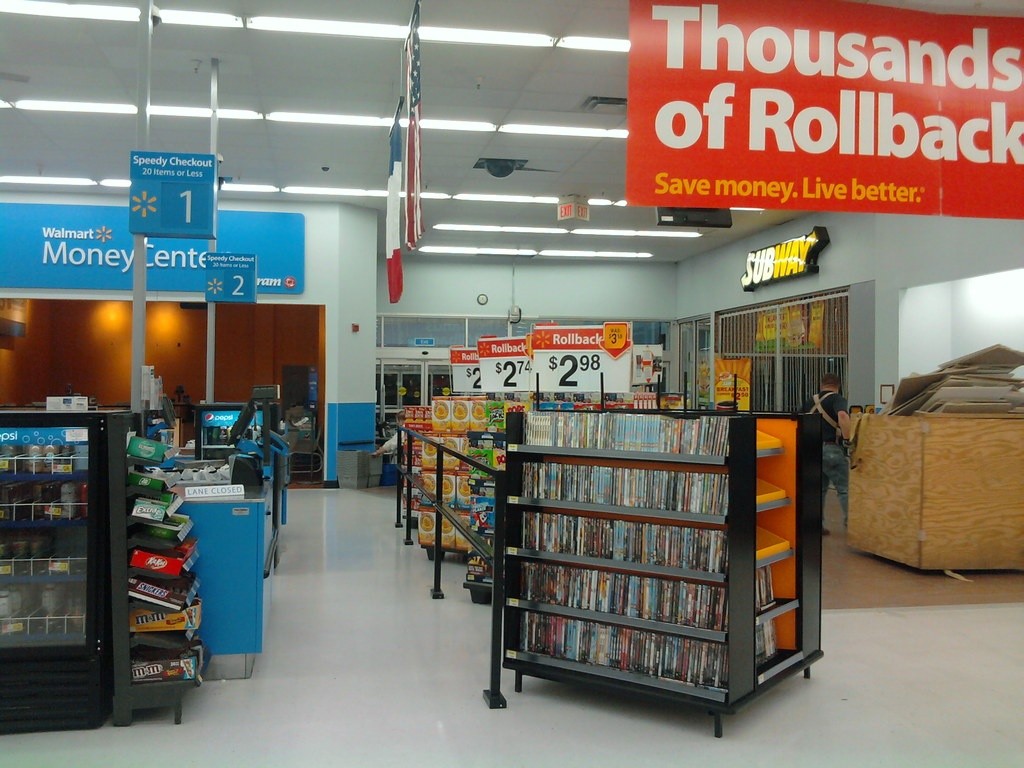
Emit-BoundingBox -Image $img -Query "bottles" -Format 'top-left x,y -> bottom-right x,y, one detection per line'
8,585 -> 21,611
42,584 -> 59,614
0,591 -> 10,617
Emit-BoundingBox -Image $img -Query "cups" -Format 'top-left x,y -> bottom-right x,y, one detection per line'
717,405 -> 738,410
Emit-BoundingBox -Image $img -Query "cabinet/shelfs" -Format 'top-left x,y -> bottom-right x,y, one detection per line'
108,409 -> 211,726
403,397 -> 480,561
429,400 -> 633,710
502,409 -> 824,738
395,406 -> 409,546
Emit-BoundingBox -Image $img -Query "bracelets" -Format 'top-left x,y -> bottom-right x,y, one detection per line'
843,439 -> 850,441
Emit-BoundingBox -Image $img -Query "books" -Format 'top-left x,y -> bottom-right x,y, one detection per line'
521,409 -> 777,691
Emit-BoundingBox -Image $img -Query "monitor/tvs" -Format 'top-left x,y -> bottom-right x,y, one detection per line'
655,207 -> 733,228
227,399 -> 257,446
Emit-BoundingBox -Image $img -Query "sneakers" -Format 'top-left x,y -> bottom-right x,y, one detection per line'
822,527 -> 832,536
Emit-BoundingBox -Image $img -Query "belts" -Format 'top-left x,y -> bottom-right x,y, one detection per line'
822,441 -> 839,445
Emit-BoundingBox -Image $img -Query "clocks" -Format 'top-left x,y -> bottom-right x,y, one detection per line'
477,294 -> 488,305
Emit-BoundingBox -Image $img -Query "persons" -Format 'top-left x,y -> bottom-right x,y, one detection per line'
798,372 -> 851,537
371,408 -> 407,458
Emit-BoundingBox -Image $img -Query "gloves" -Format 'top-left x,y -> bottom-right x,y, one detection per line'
841,438 -> 854,456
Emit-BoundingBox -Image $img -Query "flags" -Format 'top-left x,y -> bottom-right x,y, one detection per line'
385,101 -> 404,304
402,12 -> 427,251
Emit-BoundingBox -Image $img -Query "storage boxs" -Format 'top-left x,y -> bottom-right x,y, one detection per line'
367,454 -> 383,488
336,450 -> 369,489
379,464 -> 398,486
847,413 -> 1024,570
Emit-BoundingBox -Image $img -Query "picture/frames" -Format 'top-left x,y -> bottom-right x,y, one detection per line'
880,384 -> 894,404
